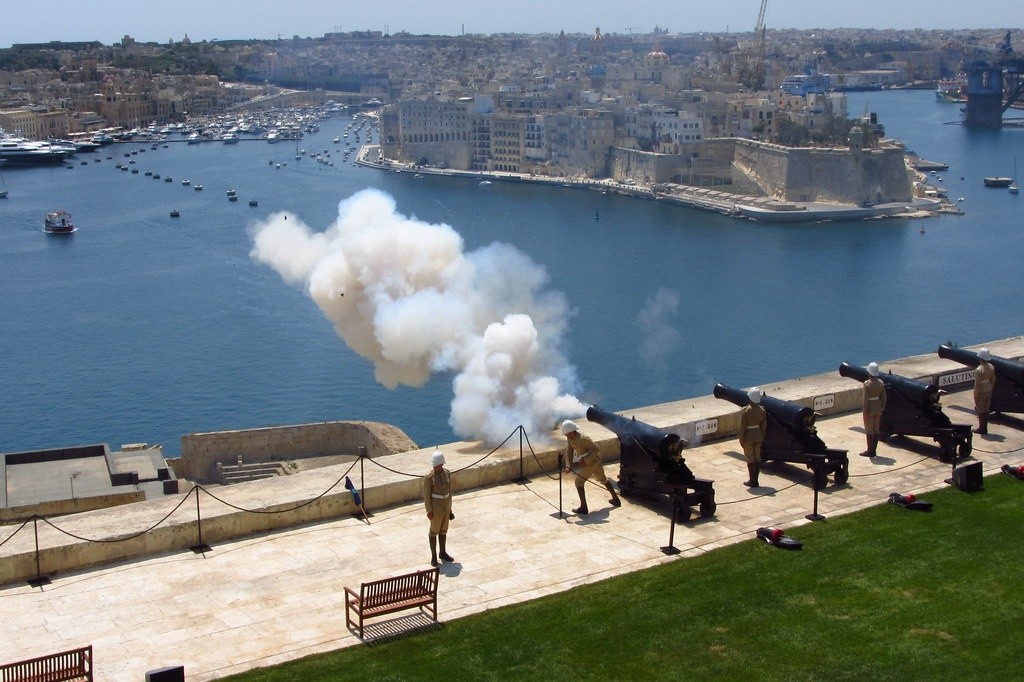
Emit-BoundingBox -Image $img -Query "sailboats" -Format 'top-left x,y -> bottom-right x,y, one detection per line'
0,173 -> 10,198
1007,155 -> 1020,193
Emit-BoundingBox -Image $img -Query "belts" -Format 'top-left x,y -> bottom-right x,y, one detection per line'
580,449 -> 592,458
981,379 -> 990,383
431,493 -> 449,500
747,426 -> 760,429
868,397 -> 880,401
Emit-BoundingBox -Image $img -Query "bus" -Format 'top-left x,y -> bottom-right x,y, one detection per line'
779,73 -> 830,98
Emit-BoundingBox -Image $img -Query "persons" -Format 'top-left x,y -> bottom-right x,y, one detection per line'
424,451 -> 454,567
561,420 -> 621,514
860,362 -> 886,456
972,348 -> 995,434
740,387 -> 766,487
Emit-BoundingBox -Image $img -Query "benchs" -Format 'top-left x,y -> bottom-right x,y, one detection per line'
345,567 -> 442,638
0,644 -> 94,682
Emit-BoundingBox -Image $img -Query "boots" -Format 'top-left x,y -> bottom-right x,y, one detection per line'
438,534 -> 454,561
743,462 -> 760,487
605,482 -> 621,506
572,486 -> 588,514
972,415 -> 988,434
430,536 -> 437,566
859,434 -> 879,457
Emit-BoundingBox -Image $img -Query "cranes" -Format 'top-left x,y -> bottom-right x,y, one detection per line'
753,0 -> 768,32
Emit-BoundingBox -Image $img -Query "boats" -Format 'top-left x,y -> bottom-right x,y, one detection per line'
0,97 -> 383,169
40,209 -> 79,236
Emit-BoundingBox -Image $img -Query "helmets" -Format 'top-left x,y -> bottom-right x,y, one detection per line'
561,420 -> 578,434
432,450 -> 445,467
867,362 -> 879,376
747,387 -> 761,403
977,348 -> 991,361
